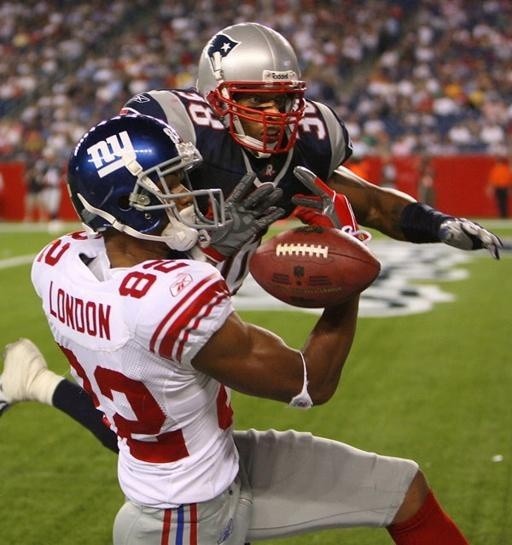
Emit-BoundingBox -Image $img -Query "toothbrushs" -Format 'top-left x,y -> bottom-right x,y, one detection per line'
247,225 -> 380,308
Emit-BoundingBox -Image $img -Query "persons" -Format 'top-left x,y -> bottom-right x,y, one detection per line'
29,114 -> 467,544
1,22 -> 502,452
1,0 -> 511,228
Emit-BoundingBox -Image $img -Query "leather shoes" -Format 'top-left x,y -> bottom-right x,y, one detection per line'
438,216 -> 503,259
291,165 -> 372,244
184,172 -> 286,267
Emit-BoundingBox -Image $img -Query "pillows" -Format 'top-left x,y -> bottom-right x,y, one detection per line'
196,22 -> 307,153
65,113 -> 225,251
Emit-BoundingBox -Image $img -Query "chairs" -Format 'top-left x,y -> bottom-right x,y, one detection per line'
0,338 -> 47,413
